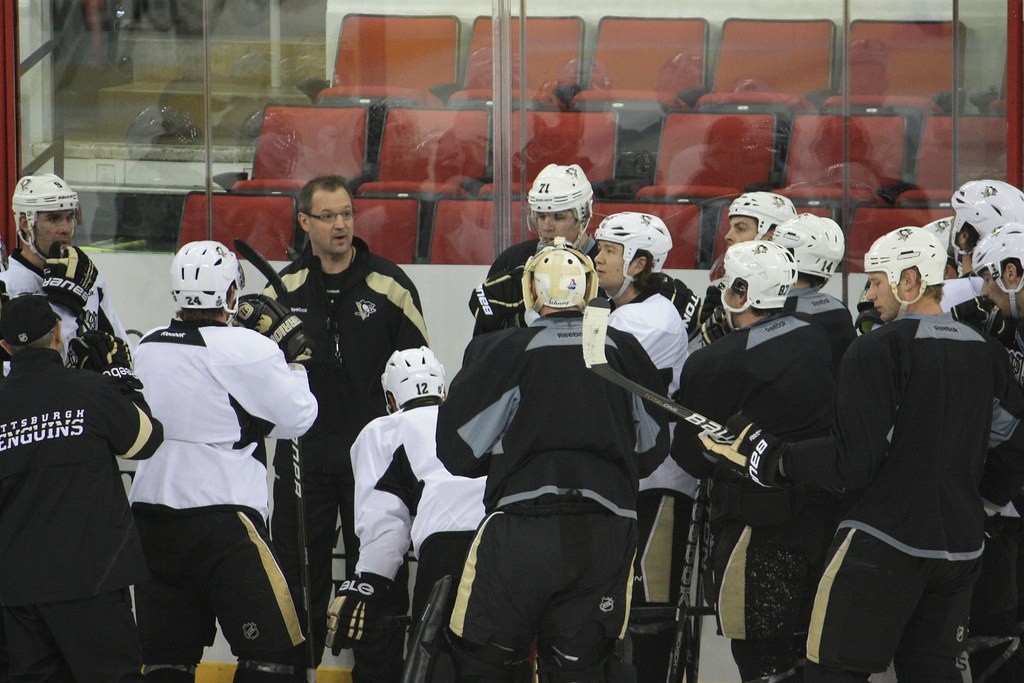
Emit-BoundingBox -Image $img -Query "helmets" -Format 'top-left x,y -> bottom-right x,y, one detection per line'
467,46 -> 527,88
521,163 -> 673,314
815,163 -> 884,203
414,130 -> 495,176
128,106 -> 200,148
855,283 -> 886,332
739,118 -> 798,160
718,191 -> 846,310
533,81 -> 587,121
352,104 -> 418,174
829,39 -> 890,70
11,173 -> 84,246
382,346 -> 446,414
864,179 -> 1024,305
169,239 -> 246,314
557,58 -> 612,113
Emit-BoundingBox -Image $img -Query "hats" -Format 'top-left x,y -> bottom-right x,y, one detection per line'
1,295 -> 64,345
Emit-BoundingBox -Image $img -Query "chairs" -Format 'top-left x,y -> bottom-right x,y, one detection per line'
174,12 -> 1011,277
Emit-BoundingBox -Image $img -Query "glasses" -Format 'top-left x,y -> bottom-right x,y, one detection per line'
305,210 -> 356,222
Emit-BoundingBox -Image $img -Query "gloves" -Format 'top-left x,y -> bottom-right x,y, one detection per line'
70,330 -> 144,391
697,415 -> 797,491
646,270 -> 702,335
324,572 -> 384,656
40,239 -> 99,314
232,294 -> 317,363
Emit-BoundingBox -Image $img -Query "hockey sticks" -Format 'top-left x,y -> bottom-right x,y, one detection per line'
292,437 -> 317,683
234,238 -> 291,312
581,296 -> 740,449
665,476 -> 709,683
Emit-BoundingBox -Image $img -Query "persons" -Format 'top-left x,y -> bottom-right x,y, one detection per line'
0,160 -> 1023,683
